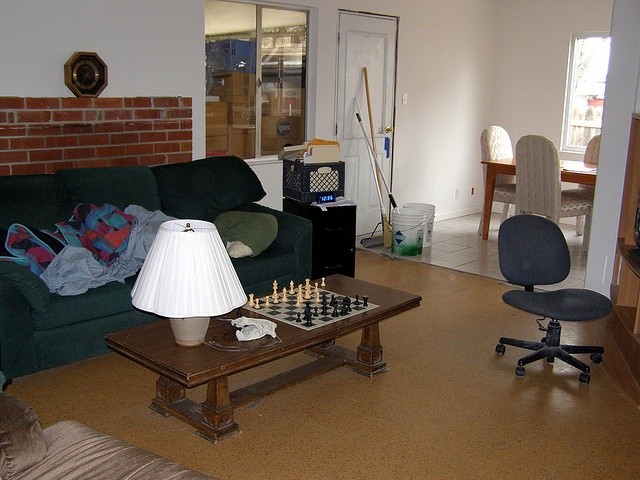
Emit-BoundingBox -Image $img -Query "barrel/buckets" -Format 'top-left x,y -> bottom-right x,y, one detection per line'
387,208 -> 426,256
402,203 -> 436,248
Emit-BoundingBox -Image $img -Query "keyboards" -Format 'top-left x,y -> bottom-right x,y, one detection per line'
628,248 -> 639,266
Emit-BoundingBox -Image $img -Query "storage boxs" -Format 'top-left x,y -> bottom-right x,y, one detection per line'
261,115 -> 303,149
208,72 -> 256,103
270,87 -> 304,115
206,136 -> 228,157
230,103 -> 255,124
228,128 -> 255,159
206,102 -> 228,136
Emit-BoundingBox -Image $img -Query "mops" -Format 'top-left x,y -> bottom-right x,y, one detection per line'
359,67 -> 392,246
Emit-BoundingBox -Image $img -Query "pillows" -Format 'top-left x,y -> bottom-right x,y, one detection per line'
0,390 -> 47,459
213,210 -> 280,258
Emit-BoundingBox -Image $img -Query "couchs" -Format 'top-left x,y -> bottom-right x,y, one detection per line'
44,418 -> 165,460
0,155 -> 311,382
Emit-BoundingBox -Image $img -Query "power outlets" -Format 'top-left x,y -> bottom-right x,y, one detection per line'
471,187 -> 475,196
455,189 -> 460,199
402,94 -> 408,105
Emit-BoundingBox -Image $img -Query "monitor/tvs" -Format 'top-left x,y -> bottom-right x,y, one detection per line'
634,207 -> 640,246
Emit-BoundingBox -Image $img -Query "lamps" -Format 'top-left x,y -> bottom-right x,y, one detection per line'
129,220 -> 251,347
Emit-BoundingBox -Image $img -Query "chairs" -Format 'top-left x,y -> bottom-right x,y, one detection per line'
581,134 -> 599,234
515,135 -> 592,240
479,126 -> 517,234
495,214 -> 612,383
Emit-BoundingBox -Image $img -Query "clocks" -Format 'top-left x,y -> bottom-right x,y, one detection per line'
63,50 -> 109,96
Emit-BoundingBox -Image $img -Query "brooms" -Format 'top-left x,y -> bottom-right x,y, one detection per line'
354,96 -> 393,248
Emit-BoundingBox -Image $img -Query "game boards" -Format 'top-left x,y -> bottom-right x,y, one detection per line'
242,285 -> 380,331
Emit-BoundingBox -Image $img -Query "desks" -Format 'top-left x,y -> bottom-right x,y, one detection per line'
281,196 -> 358,275
